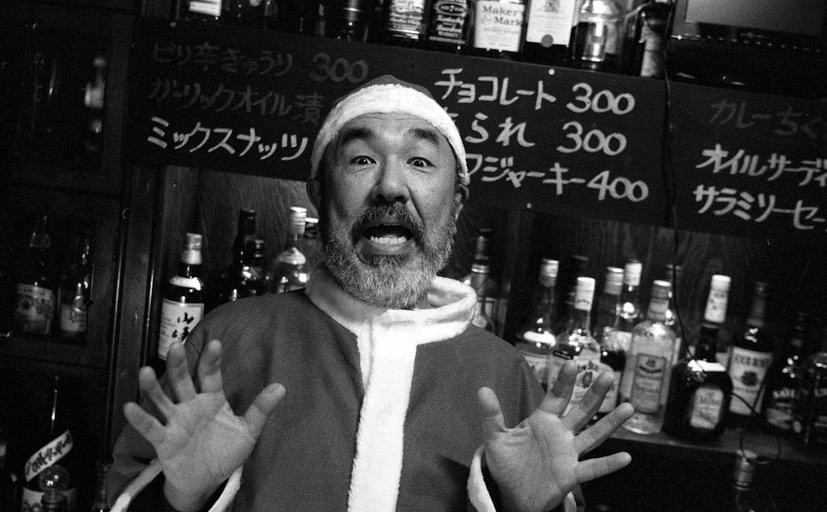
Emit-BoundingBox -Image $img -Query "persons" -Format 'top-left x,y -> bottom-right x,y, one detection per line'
100,74 -> 637,512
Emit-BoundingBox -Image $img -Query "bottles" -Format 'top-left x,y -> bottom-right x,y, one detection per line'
724,449 -> 759,508
2,200 -> 90,370
0,353 -> 82,510
191,0 -> 664,79
460,228 -> 826,438
156,198 -> 324,373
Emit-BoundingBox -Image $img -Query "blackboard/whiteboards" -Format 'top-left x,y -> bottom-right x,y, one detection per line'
140,25 -> 827,237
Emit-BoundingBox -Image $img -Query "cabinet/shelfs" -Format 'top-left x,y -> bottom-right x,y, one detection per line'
0,0 -> 826,505
